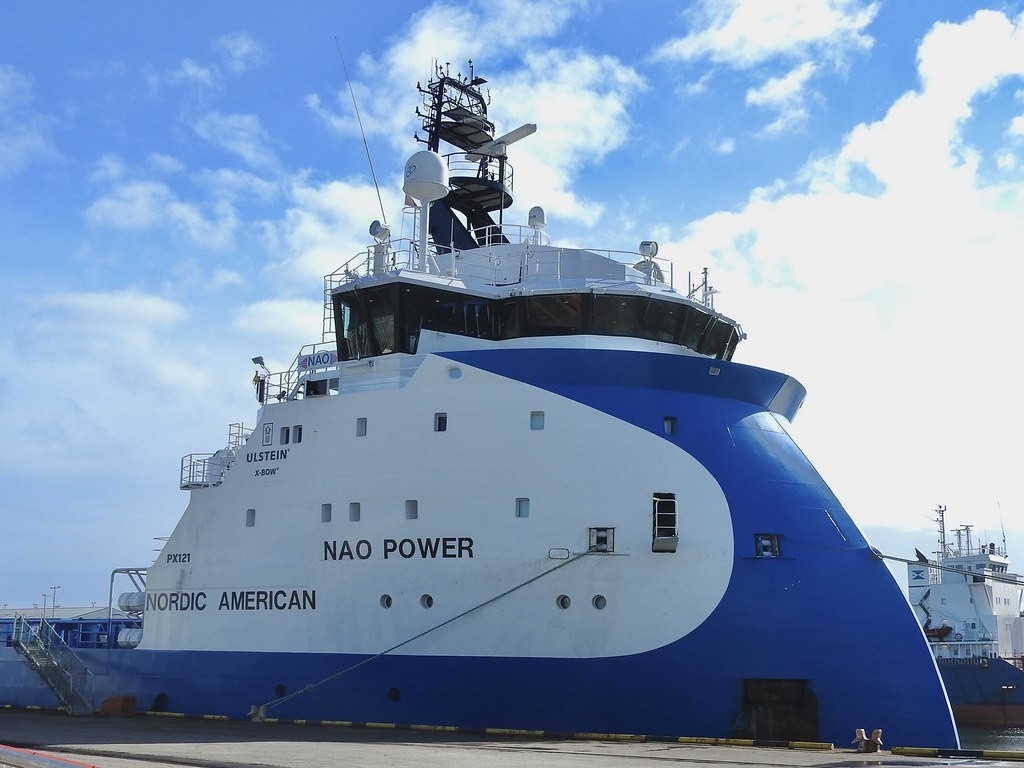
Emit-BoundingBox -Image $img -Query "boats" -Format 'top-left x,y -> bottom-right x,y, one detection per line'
871,503 -> 1024,732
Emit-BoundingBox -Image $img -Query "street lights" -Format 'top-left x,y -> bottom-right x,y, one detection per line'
49,585 -> 61,617
42,593 -> 49,617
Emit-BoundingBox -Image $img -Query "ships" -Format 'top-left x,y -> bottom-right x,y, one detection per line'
0,49 -> 967,754
